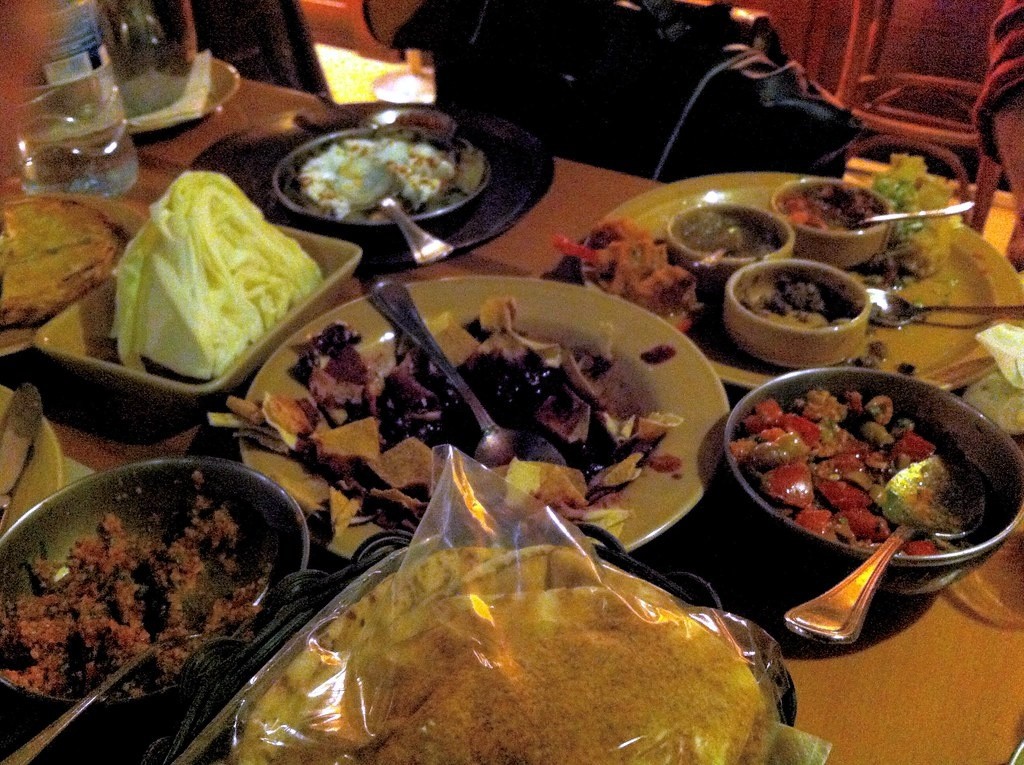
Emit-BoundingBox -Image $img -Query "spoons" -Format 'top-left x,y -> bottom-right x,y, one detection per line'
780,453 -> 988,644
367,277 -> 570,489
863,283 -> 1024,329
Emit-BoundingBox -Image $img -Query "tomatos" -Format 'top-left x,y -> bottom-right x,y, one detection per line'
730,397 -> 941,559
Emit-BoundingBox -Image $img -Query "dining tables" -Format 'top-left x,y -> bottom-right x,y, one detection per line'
0,56 -> 1024,765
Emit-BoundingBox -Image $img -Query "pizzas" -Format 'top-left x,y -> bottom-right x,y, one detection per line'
0,196 -> 121,329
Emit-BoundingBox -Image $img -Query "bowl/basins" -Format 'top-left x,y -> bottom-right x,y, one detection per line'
725,368 -> 1024,597
30,223 -> 361,404
769,176 -> 895,270
273,122 -> 492,226
720,258 -> 870,368
0,456 -> 311,713
667,204 -> 796,290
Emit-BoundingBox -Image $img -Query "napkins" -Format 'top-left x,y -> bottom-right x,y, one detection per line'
31,45 -> 213,135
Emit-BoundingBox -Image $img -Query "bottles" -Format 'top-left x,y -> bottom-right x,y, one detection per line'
0,0 -> 137,199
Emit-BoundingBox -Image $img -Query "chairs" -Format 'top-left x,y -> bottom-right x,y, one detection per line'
391,0 -> 849,197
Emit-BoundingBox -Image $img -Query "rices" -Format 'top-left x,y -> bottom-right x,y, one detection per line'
0,471 -> 271,700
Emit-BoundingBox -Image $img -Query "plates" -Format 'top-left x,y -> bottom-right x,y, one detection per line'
0,384 -> 64,560
0,190 -> 147,358
121,58 -> 241,133
577,170 -> 1023,396
188,102 -> 553,272
235,274 -> 731,580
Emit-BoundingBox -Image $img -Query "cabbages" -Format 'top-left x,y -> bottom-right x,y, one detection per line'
109,169 -> 325,380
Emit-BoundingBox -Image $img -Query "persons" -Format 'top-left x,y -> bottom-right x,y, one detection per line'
972,0 -> 1024,274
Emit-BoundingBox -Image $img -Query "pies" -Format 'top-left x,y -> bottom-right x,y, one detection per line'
223,541 -> 776,765
